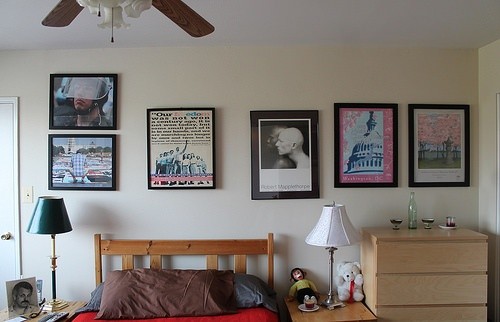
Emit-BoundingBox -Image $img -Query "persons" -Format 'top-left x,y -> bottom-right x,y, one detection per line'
8,282 -> 40,315
262,126 -> 310,169
154,140 -> 211,185
67,77 -> 109,128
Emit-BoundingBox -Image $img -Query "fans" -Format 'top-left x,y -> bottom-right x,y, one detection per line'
41,0 -> 215,40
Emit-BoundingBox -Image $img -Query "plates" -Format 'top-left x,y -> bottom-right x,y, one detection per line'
438,223 -> 459,229
298,304 -> 319,312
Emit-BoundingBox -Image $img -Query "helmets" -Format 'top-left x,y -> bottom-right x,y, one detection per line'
72,77 -> 112,103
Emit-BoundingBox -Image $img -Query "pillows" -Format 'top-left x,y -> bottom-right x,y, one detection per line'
94,267 -> 242,320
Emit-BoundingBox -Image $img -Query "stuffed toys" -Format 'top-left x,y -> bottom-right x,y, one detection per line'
336,262 -> 365,302
288,268 -> 319,305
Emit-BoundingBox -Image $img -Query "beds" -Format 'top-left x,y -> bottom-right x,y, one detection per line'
70,233 -> 281,322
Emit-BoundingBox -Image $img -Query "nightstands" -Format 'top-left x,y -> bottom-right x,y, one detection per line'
284,295 -> 378,322
0,300 -> 88,322
359,224 -> 489,322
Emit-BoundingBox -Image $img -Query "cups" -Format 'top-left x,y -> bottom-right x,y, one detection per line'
304,299 -> 315,309
446,216 -> 456,227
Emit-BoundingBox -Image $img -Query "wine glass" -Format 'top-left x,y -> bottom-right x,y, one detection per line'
390,216 -> 403,230
422,217 -> 435,229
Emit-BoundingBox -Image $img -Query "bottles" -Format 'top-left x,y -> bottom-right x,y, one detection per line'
407,191 -> 417,229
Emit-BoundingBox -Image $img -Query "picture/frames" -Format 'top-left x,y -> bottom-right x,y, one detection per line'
407,103 -> 470,187
249,108 -> 320,200
333,102 -> 400,189
48,133 -> 116,191
49,73 -> 117,130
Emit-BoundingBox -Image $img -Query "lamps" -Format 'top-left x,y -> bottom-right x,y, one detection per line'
26,196 -> 74,309
304,200 -> 362,310
76,0 -> 152,44
